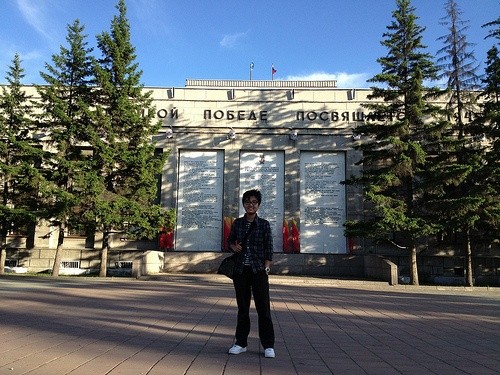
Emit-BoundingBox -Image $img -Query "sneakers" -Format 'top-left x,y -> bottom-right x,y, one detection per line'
265,348 -> 275,357
229,345 -> 247,354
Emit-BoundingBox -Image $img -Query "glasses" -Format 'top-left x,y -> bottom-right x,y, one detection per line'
244,199 -> 259,205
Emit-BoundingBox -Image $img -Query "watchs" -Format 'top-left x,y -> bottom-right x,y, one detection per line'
264,267 -> 271,272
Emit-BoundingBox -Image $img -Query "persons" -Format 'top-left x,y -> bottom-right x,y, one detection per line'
227,190 -> 277,359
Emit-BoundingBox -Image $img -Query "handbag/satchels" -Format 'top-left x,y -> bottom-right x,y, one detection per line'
217,254 -> 236,278
